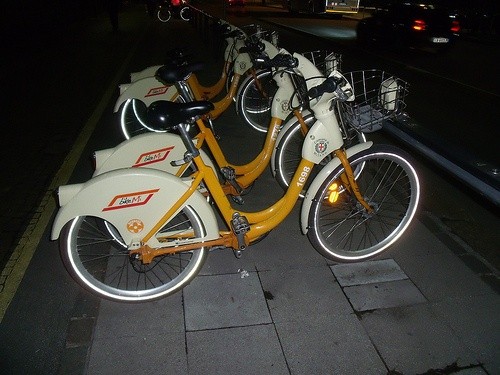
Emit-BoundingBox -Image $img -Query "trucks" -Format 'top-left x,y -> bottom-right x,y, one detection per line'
287,0 -> 360,18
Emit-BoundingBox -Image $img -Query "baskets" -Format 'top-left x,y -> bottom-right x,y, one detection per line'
300,49 -> 343,77
341,69 -> 411,135
239,24 -> 279,51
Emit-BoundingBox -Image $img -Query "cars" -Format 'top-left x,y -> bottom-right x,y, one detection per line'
356,2 -> 468,55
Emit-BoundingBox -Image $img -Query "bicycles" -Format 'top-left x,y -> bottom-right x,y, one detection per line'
158,2 -> 194,22
50,24 -> 427,303
